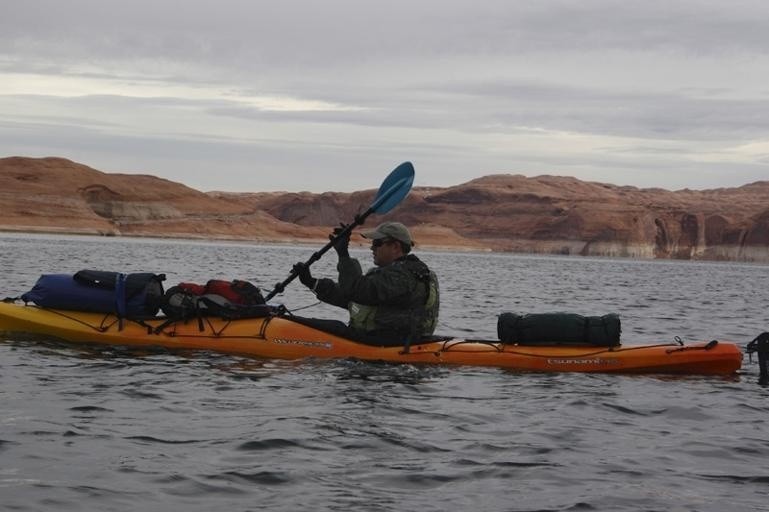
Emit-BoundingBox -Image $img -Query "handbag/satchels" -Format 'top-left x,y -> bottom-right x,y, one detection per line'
163,280 -> 266,320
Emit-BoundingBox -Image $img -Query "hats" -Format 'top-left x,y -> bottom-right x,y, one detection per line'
360,221 -> 413,248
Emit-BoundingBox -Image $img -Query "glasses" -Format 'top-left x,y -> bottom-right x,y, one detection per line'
372,236 -> 394,248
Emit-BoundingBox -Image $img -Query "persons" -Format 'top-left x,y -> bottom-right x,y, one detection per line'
290,221 -> 439,348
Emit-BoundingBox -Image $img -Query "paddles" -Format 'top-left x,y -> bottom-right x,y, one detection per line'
264,161 -> 415,303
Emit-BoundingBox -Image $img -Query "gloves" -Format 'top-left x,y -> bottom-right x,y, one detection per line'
289,262 -> 317,289
329,221 -> 352,256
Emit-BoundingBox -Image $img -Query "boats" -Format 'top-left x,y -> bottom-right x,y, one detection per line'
1,300 -> 743,379
